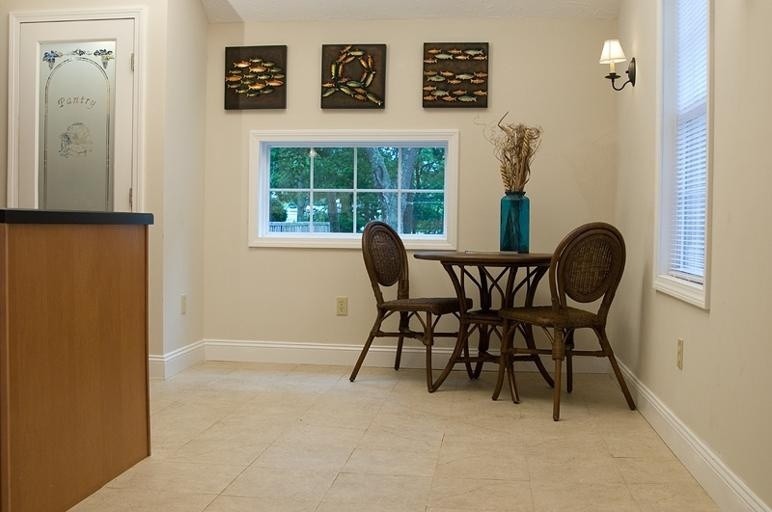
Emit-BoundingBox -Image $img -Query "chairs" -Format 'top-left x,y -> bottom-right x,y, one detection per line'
498,222 -> 637,419
349,221 -> 473,391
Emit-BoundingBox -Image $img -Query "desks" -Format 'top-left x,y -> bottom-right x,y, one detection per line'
413,250 -> 554,401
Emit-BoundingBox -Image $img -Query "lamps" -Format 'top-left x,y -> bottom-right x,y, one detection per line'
597,37 -> 635,91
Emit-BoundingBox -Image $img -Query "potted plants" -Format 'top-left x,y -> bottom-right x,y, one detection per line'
471,110 -> 545,253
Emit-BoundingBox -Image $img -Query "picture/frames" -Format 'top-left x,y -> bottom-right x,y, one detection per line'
223,41 -> 490,110
